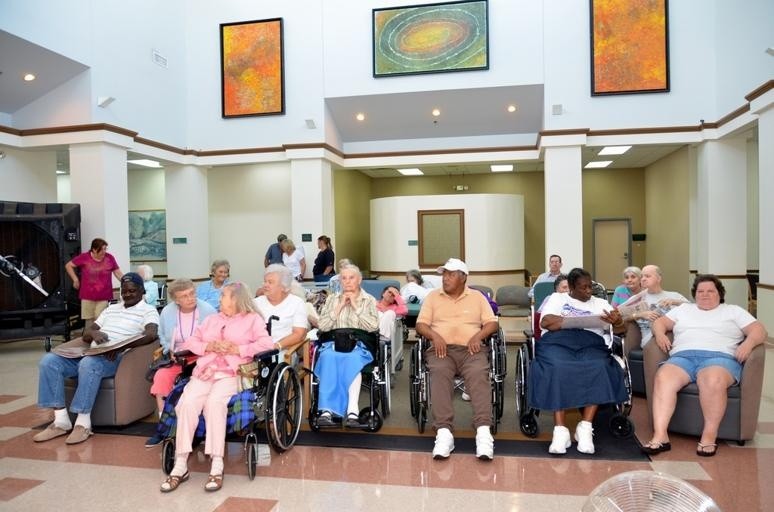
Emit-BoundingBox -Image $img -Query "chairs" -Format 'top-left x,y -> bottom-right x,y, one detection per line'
283,338 -> 311,433
468,285 -> 531,317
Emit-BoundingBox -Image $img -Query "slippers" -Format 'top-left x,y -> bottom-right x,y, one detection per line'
696,440 -> 721,458
642,439 -> 673,455
34,425 -> 68,442
67,427 -> 93,444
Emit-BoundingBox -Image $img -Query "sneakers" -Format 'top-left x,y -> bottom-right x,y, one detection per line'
475,435 -> 495,461
431,431 -> 455,460
145,433 -> 165,448
575,424 -> 597,456
549,431 -> 572,456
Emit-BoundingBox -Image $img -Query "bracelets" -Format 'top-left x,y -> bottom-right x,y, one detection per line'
613,320 -> 626,327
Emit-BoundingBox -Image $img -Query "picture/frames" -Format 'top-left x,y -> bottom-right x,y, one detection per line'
218,17 -> 285,119
127,209 -> 166,262
372,0 -> 489,78
590,0 -> 670,97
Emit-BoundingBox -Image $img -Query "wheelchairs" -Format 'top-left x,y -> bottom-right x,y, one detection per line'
305,333 -> 393,435
408,291 -> 508,436
107,277 -> 169,316
324,280 -> 405,390
158,314 -> 304,482
514,281 -> 635,438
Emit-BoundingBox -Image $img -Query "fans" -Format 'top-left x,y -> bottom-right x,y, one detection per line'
582,470 -> 722,512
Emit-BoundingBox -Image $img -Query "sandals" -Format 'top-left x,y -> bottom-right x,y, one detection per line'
205,472 -> 226,492
160,469 -> 191,493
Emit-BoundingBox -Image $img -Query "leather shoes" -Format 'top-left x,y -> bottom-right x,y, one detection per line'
317,410 -> 332,426
345,412 -> 360,427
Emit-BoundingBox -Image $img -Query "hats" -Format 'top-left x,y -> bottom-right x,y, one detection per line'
120,272 -> 147,296
436,258 -> 470,275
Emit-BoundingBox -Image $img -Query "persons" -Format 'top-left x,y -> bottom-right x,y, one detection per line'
527,269 -> 627,454
250,264 -> 309,365
135,265 -> 159,304
328,258 -> 349,292
538,275 -> 569,315
196,259 -> 232,310
401,271 -> 430,304
315,263 -> 379,427
640,274 -> 767,456
145,279 -> 216,450
528,254 -> 563,298
263,234 -> 288,267
618,265 -> 691,347
281,240 -> 307,282
64,237 -> 124,334
376,285 -> 408,343
611,266 -> 642,310
313,236 -> 335,282
33,271 -> 159,445
160,281 -> 275,493
415,257 -> 498,461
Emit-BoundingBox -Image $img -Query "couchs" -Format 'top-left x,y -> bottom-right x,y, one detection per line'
624,321 -> 646,394
643,331 -> 765,446
64,337 -> 160,425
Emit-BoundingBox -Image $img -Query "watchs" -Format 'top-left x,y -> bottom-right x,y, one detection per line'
632,313 -> 637,321
